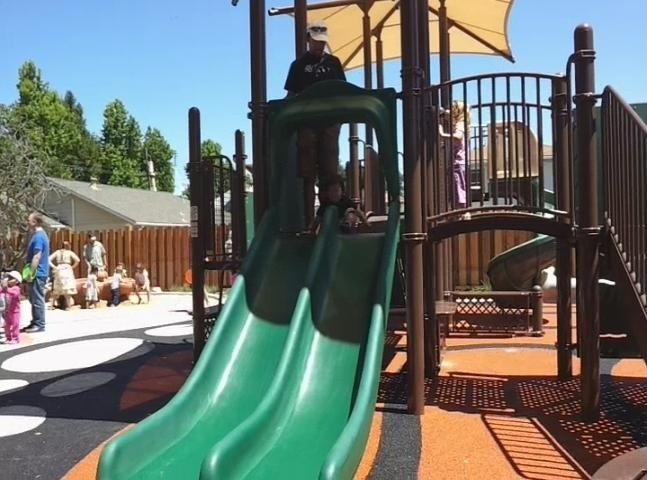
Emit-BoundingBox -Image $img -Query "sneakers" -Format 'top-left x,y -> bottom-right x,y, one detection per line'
0,338 -> 19,344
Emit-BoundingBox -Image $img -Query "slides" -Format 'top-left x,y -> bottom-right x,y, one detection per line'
95,80 -> 399,476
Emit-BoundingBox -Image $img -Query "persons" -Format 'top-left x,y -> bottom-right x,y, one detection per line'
0,270 -> 21,346
438,98 -> 472,222
301,172 -> 371,233
22,211 -> 50,333
82,231 -> 109,279
113,262 -> 127,277
134,261 -> 150,304
49,240 -> 81,311
107,266 -> 123,307
84,263 -> 100,309
282,19 -> 346,232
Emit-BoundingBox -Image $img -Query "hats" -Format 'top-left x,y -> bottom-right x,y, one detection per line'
6,270 -> 23,284
21,264 -> 37,284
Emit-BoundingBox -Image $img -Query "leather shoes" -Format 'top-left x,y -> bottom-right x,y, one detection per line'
22,324 -> 45,333
